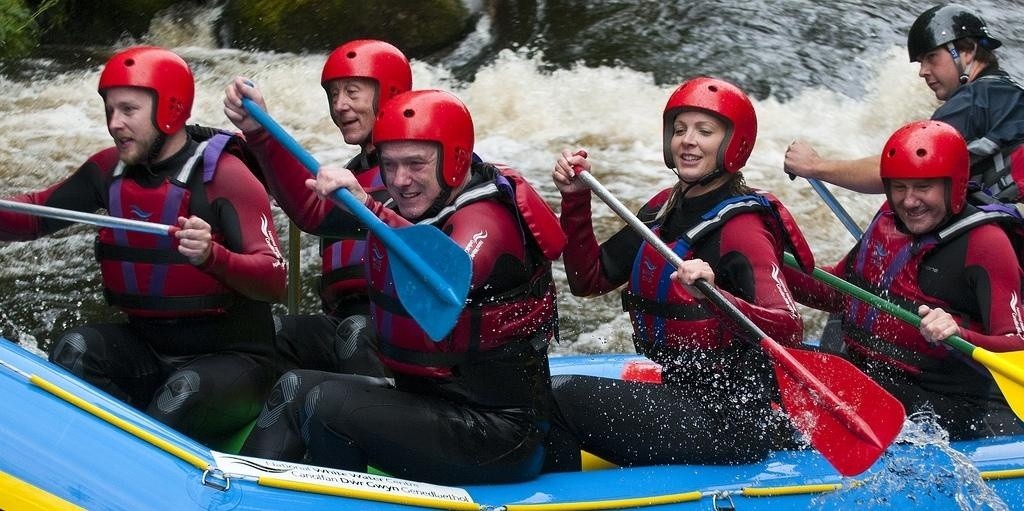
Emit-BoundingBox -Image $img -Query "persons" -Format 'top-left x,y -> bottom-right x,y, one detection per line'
0,45 -> 287,443
779,120 -> 1024,438
237,87 -> 557,486
550,76 -> 802,472
272,37 -> 414,381
785,2 -> 1024,209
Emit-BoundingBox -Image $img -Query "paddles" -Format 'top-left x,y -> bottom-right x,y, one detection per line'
569,150 -> 905,476
238,82 -> 472,343
782,254 -> 1024,422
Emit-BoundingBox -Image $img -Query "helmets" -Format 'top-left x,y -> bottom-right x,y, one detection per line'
907,4 -> 1003,61
98,48 -> 195,137
879,119 -> 971,215
662,76 -> 759,174
322,38 -> 413,115
373,89 -> 474,190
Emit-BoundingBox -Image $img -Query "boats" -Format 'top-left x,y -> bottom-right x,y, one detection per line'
0,338 -> 1023,509
